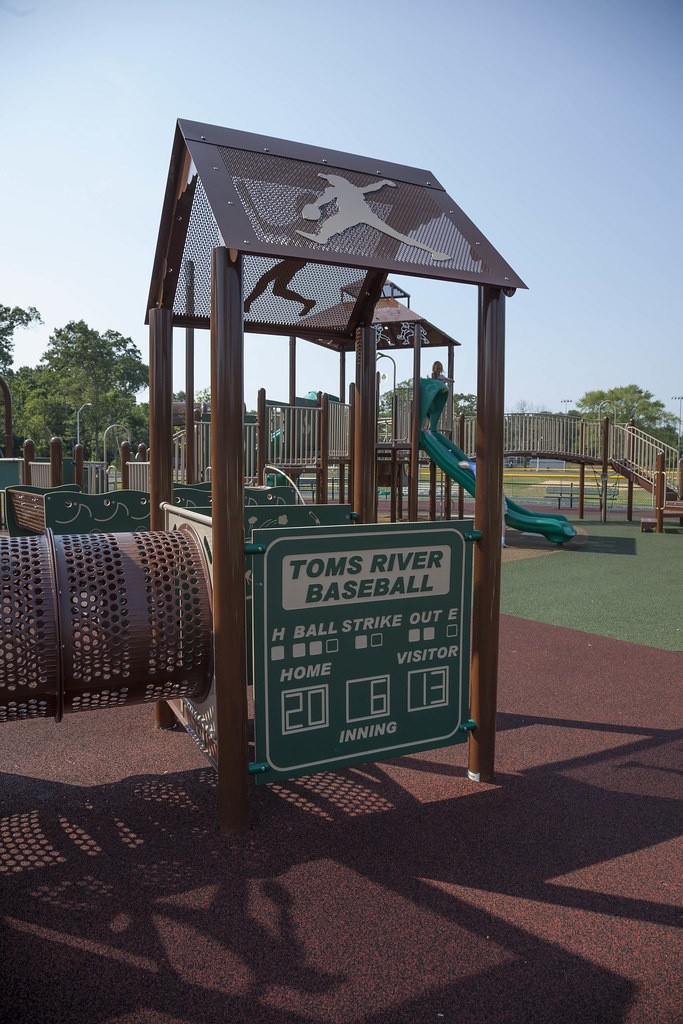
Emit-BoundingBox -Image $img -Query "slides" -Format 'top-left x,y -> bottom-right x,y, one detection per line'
421,430 -> 578,544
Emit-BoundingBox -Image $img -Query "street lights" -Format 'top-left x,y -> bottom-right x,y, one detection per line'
537,437 -> 543,472
670,397 -> 683,457
560,399 -> 574,416
77,402 -> 91,444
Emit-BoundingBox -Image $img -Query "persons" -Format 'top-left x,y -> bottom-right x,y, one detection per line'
426,361 -> 455,384
423,416 -> 437,441
457,460 -> 509,547
